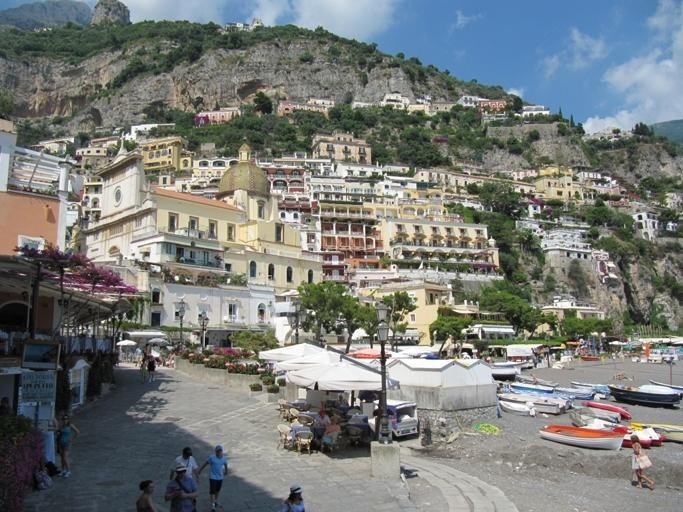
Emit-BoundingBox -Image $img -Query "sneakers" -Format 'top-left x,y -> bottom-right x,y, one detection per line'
58,469 -> 71,477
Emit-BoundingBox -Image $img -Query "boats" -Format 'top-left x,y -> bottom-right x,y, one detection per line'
582,400 -> 632,419
649,353 -> 679,364
538,423 -> 624,451
571,405 -> 623,424
490,360 -> 595,416
568,410 -> 662,449
581,355 -> 600,362
648,378 -> 683,397
607,383 -> 680,408
572,381 -> 612,400
630,421 -> 683,444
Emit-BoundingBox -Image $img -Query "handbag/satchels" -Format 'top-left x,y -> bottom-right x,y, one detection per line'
636,455 -> 653,470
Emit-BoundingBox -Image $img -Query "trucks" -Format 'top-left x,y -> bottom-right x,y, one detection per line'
372,399 -> 420,437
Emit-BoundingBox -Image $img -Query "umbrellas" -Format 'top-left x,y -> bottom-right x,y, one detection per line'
149,337 -> 170,350
115,340 -> 134,357
258,342 -> 400,391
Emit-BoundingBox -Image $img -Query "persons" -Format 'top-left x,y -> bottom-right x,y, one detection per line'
279,485 -> 305,512
164,463 -> 200,512
170,447 -> 200,483
139,359 -> 149,384
136,480 -> 158,512
55,414 -> 80,478
197,445 -> 228,512
324,415 -> 341,435
631,435 -> 655,490
147,356 -> 156,384
44,416 -> 59,464
315,410 -> 331,425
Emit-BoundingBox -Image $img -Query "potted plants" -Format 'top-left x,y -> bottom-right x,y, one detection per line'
174,343 -> 288,403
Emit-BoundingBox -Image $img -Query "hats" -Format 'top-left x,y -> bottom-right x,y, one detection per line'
175,462 -> 186,472
290,485 -> 303,493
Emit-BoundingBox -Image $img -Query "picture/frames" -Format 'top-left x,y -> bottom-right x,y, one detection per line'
20,340 -> 61,371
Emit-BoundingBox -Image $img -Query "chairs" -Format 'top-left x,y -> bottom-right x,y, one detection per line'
274,395 -> 372,456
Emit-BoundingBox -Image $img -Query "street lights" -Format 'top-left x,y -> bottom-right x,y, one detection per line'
177,298 -> 186,355
287,301 -> 305,344
374,300 -> 393,444
198,309 -> 210,351
